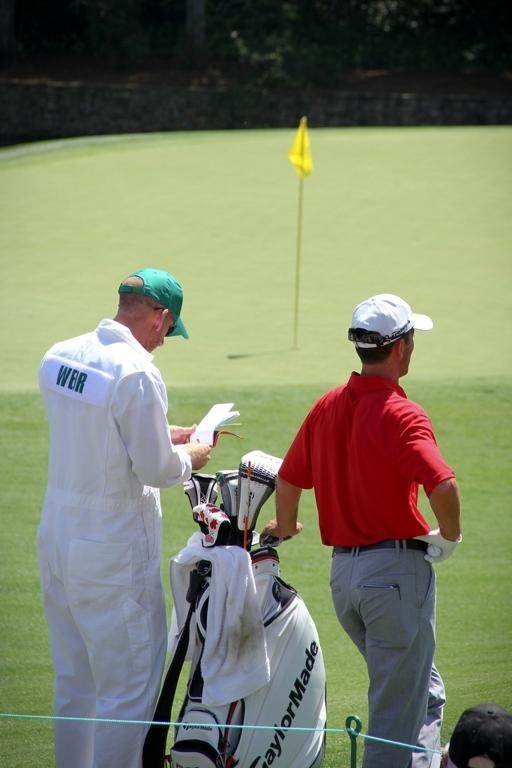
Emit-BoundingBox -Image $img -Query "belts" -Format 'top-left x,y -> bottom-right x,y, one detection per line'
332,538 -> 430,554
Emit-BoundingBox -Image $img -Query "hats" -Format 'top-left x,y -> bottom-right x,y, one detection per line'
351,292 -> 433,349
118,267 -> 190,339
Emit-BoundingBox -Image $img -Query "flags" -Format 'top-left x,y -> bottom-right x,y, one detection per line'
288,118 -> 312,175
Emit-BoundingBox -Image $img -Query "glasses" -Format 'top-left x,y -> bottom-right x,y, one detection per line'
348,328 -> 404,344
153,307 -> 175,337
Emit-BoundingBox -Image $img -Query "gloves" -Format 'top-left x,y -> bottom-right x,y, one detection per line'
410,527 -> 463,564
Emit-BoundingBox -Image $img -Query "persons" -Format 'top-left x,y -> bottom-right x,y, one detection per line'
38,268 -> 212,768
259,293 -> 463,767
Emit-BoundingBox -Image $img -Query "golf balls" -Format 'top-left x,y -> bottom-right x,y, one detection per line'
426,543 -> 443,559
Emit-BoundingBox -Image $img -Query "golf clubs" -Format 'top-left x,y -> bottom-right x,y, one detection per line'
183,449 -> 284,549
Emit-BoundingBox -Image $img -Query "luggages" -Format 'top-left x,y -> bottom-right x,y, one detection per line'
141,530 -> 327,768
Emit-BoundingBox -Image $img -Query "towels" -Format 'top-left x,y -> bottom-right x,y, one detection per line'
168,530 -> 271,707
